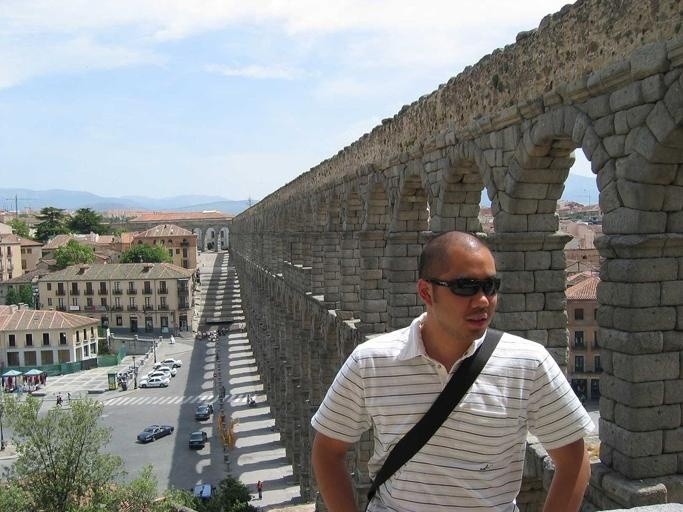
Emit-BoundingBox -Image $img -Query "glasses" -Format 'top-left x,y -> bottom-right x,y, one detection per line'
429,276 -> 501,297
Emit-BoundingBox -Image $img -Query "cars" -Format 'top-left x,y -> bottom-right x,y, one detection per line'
136,424 -> 173,442
195,404 -> 211,420
138,358 -> 181,388
188,431 -> 207,449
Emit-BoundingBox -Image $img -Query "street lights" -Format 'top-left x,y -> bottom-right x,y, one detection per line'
131,356 -> 136,389
32,288 -> 38,310
152,336 -> 156,363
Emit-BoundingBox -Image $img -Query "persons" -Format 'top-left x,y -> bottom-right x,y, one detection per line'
255,479 -> 264,500
56,396 -> 62,407
67,393 -> 71,403
311,230 -> 597,510
57,392 -> 62,403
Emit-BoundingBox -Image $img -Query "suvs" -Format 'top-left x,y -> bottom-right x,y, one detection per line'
190,483 -> 216,501
